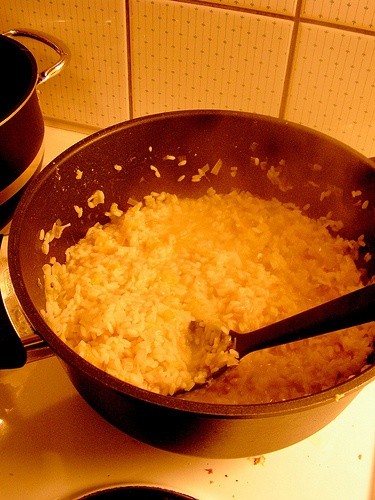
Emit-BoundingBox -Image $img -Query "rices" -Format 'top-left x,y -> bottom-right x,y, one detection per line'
38,152 -> 375,406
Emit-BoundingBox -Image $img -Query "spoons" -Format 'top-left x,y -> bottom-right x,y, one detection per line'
170,282 -> 374,395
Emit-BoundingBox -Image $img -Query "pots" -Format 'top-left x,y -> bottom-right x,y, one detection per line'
0,110 -> 374,459
0,27 -> 69,238
